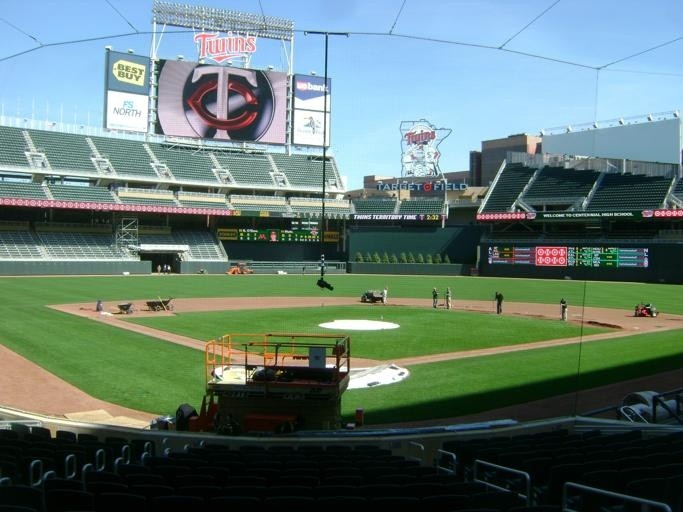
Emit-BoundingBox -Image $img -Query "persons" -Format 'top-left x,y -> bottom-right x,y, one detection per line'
214,404 -> 240,435
156,264 -> 171,274
495,292 -> 503,314
634,302 -> 660,317
281,415 -> 297,433
444,287 -> 452,309
432,288 -> 440,308
382,287 -> 388,304
560,298 -> 568,320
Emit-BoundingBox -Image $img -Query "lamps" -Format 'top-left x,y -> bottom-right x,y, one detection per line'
177,55 -> 184,59
268,65 -> 274,69
311,72 -> 316,75
105,46 -> 111,51
128,49 -> 134,53
227,59 -> 232,65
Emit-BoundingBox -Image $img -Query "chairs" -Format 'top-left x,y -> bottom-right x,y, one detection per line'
482,163 -> 683,212
